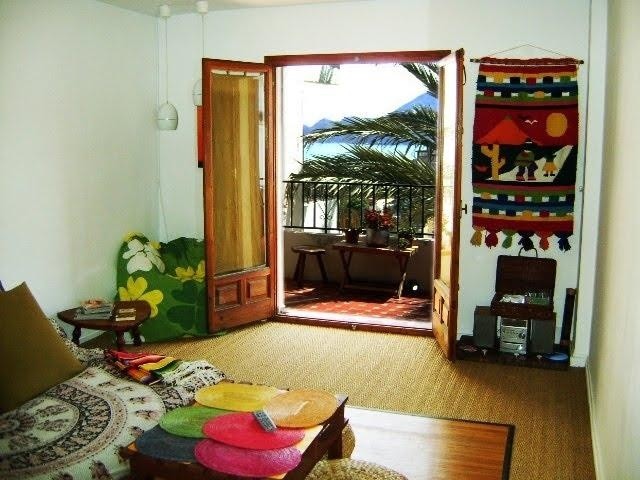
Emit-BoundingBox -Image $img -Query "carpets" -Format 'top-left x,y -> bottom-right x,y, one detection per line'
8,317 -> 227,480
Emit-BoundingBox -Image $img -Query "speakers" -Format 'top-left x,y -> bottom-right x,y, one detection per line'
473,306 -> 497,348
531,320 -> 556,354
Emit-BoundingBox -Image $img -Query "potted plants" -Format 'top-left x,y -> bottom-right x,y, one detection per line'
338,213 -> 363,243
397,217 -> 416,247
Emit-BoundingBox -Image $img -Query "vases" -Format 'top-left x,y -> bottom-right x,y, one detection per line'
367,228 -> 389,246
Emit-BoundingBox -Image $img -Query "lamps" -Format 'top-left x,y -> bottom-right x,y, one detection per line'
155,4 -> 178,129
192,1 -> 208,105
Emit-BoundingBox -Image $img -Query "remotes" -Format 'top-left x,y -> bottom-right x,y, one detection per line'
253,410 -> 276,433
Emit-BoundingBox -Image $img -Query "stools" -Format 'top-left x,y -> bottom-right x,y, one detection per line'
293,246 -> 328,285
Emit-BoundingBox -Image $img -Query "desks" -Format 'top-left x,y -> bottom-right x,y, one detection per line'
333,239 -> 418,299
121,379 -> 348,480
55,301 -> 151,350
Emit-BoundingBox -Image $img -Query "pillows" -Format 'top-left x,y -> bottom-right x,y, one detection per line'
111,232 -> 228,346
0,281 -> 83,413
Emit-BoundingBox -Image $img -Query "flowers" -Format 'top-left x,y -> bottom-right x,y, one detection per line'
365,209 -> 396,230
